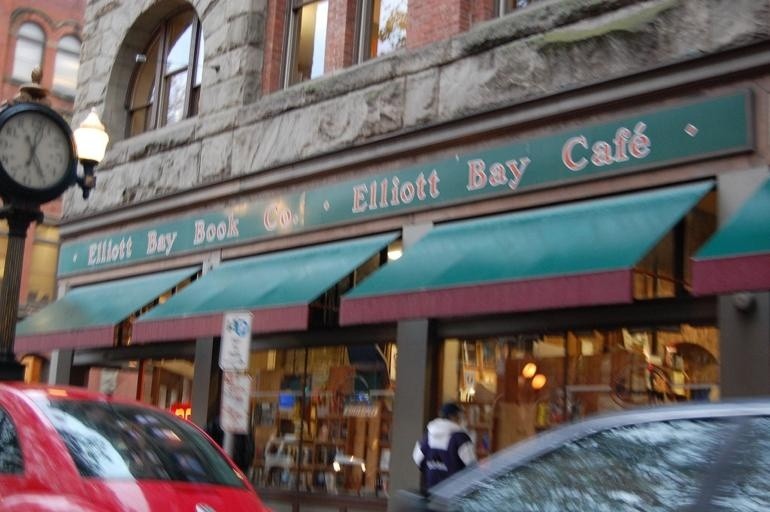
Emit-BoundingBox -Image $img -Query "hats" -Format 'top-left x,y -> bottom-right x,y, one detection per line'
441,403 -> 462,415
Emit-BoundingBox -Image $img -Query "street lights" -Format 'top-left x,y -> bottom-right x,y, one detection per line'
0,66 -> 111,382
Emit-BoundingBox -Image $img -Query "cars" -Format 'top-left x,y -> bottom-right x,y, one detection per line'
1,380 -> 272,512
397,399 -> 768,509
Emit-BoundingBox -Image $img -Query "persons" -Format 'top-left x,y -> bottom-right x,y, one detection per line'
412,401 -> 476,496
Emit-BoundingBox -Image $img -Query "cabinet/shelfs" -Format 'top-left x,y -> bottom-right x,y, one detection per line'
272,404 -> 346,495
456,399 -> 497,459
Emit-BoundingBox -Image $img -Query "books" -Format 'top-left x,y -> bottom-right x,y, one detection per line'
249,390 -> 391,497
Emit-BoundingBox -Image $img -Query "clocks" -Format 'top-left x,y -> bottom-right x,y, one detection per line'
0,101 -> 77,205
